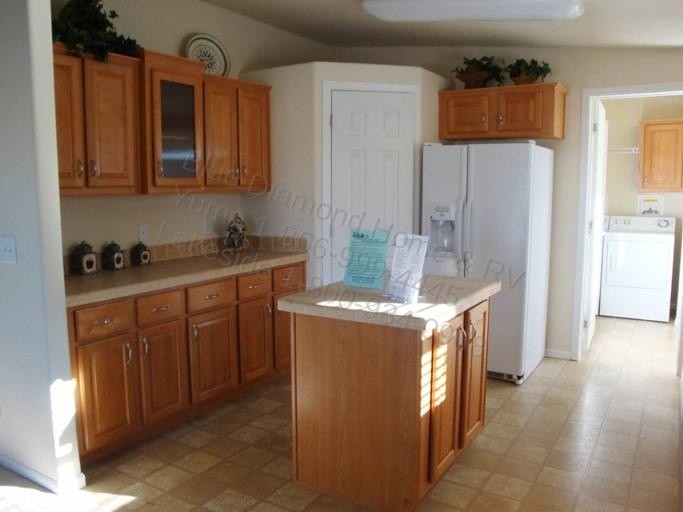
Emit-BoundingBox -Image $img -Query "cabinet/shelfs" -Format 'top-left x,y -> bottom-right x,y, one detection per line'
290,299 -> 491,512
185,274 -> 239,417
203,72 -> 272,193
639,119 -> 683,192
438,82 -> 568,141
66,285 -> 188,465
53,40 -> 141,197
137,48 -> 206,194
238,261 -> 306,396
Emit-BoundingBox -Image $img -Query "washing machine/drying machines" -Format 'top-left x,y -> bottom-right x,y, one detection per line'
598,215 -> 676,324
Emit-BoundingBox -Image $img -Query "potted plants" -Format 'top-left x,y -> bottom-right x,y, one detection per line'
452,56 -> 502,89
504,58 -> 551,84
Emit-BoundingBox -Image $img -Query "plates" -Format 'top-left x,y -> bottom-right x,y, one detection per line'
185,31 -> 231,77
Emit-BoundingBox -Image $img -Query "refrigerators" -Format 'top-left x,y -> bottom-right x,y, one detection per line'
421,139 -> 555,386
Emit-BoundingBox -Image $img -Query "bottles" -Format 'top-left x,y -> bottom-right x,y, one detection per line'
73,240 -> 98,275
131,240 -> 151,266
102,239 -> 125,271
